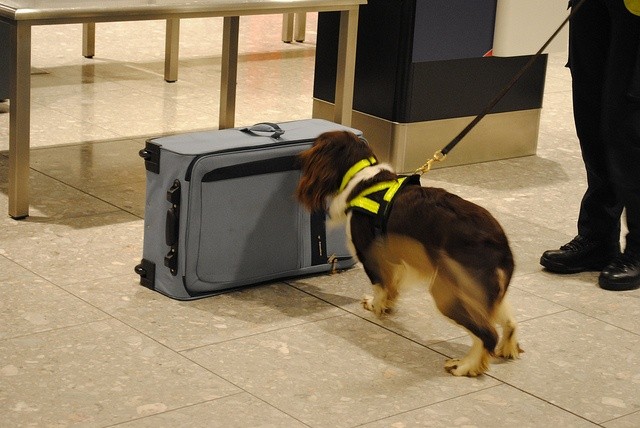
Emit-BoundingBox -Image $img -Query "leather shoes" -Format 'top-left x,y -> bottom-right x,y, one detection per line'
599,254 -> 640,291
540,235 -> 621,274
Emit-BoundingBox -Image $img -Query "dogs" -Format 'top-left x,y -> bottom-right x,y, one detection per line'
292,130 -> 526,378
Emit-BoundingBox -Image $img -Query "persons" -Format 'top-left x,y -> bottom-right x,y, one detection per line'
540,0 -> 639,291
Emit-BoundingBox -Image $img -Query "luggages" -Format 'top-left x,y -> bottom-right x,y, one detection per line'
134,118 -> 369,301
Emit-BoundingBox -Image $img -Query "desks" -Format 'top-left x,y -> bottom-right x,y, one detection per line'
1,1 -> 369,221
